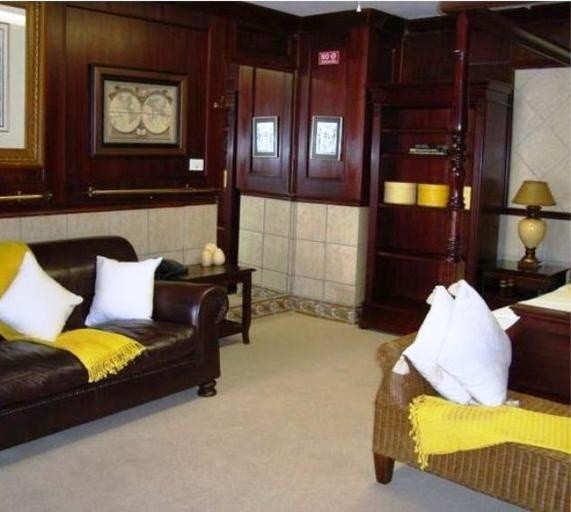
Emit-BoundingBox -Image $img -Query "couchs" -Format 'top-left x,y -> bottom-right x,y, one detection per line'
371,303 -> 571,512
0,236 -> 229,452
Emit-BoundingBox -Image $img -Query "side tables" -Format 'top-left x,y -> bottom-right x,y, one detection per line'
481,259 -> 570,311
167,263 -> 257,344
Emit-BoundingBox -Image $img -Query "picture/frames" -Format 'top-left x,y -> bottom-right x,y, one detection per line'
87,63 -> 191,156
309,116 -> 342,162
251,116 -> 279,159
0,1 -> 46,170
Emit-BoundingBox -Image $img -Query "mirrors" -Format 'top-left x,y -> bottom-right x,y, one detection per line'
225,25 -> 300,326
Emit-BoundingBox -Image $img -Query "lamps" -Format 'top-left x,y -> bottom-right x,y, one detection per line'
512,180 -> 557,270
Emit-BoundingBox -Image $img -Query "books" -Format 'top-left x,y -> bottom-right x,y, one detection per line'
408,142 -> 447,155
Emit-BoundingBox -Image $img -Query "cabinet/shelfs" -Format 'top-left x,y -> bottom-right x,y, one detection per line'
359,75 -> 514,335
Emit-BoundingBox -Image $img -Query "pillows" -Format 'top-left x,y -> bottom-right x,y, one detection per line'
438,280 -> 513,407
0,248 -> 84,340
85,255 -> 164,327
403,286 -> 474,404
